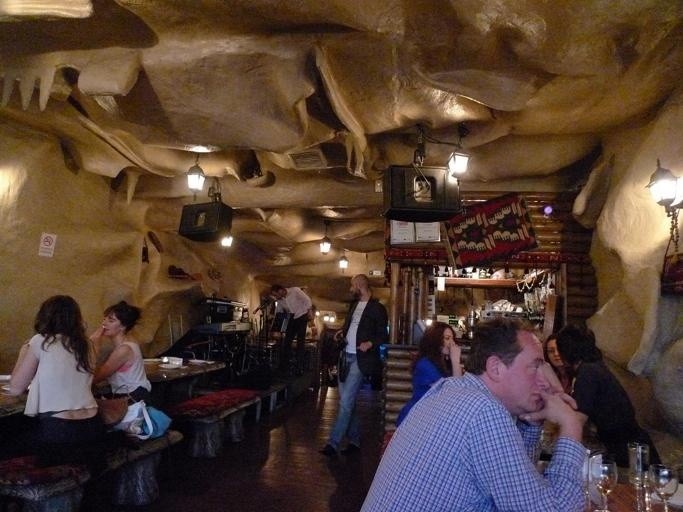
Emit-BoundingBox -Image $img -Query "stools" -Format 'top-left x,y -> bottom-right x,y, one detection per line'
264,383 -> 289,414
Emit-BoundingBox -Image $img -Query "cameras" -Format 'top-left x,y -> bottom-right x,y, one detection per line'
335,337 -> 346,351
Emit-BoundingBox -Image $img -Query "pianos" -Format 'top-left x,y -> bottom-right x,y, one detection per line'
192,321 -> 253,336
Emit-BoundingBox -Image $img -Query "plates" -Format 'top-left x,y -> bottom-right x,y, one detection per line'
191,359 -> 216,365
158,364 -> 181,371
143,359 -> 161,365
0,374 -> 12,380
652,484 -> 683,507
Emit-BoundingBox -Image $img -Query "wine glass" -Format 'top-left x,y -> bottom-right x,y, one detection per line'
653,465 -> 678,512
627,445 -> 649,507
593,462 -> 618,511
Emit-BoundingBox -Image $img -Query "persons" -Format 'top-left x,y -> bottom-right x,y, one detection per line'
268,285 -> 312,376
543,335 -> 574,396
360,317 -> 588,511
88,300 -> 153,421
556,322 -> 664,473
395,323 -> 461,426
319,273 -> 388,454
8,294 -> 100,446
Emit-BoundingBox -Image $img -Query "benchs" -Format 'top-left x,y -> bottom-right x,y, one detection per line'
0,426 -> 184,512
167,387 -> 263,458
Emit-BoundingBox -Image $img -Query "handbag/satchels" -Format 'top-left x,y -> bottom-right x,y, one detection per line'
95,394 -> 175,442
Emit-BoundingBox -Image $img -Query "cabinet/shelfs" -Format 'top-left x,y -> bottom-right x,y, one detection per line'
427,263 -> 556,334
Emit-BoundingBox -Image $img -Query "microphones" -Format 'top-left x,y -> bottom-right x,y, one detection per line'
253,297 -> 270,314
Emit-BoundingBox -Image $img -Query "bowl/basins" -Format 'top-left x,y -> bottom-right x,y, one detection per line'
163,357 -> 183,367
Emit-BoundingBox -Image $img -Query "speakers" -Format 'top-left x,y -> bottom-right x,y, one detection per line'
178,203 -> 234,243
383,165 -> 463,220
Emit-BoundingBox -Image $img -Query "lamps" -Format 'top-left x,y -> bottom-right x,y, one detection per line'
339,251 -> 348,274
319,217 -> 332,254
646,156 -> 682,214
185,151 -> 206,192
445,122 -> 470,186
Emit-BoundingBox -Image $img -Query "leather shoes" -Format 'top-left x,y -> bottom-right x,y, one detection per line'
318,444 -> 337,457
341,445 -> 360,456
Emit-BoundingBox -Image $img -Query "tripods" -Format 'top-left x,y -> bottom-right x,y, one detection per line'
260,306 -> 274,348
